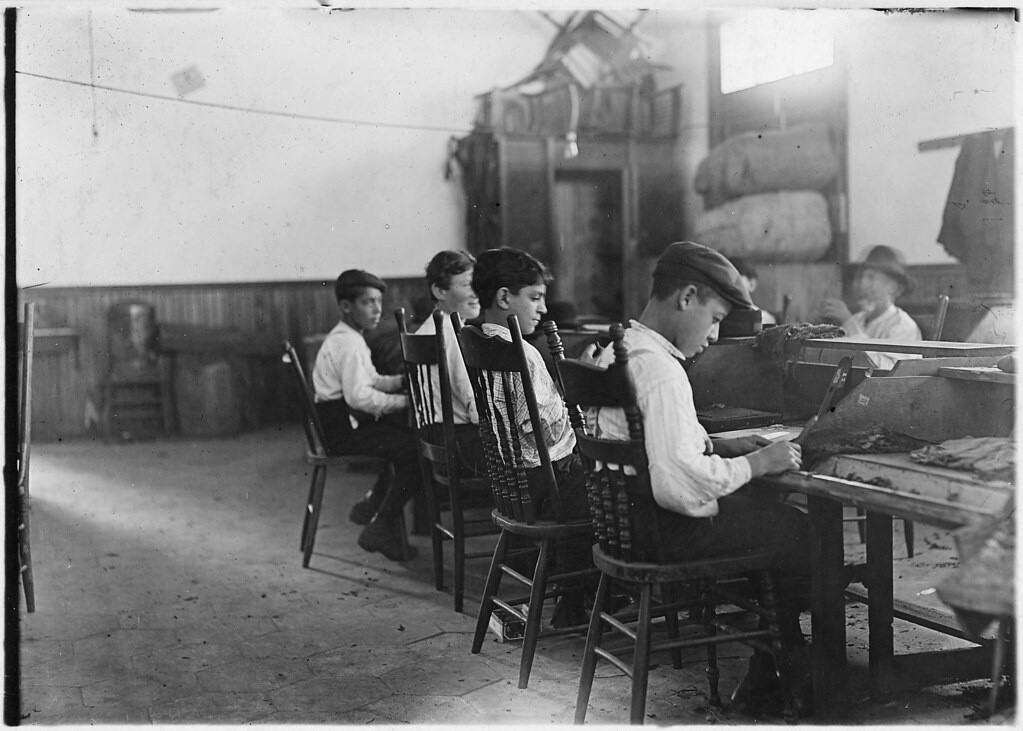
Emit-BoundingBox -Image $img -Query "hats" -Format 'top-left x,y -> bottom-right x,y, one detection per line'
335,269 -> 386,293
652,241 -> 760,312
841,245 -> 916,301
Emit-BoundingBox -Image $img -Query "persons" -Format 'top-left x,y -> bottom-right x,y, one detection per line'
473,245 -> 635,638
579,242 -> 870,722
312,269 -> 420,561
410,249 -> 585,580
820,246 -> 924,371
728,254 -> 778,329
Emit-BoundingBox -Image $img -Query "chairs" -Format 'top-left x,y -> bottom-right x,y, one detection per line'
473,9 -> 686,160
895,294 -> 949,358
394,307 -> 800,725
280,340 -> 410,568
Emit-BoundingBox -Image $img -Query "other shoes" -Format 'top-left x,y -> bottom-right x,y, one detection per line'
348,496 -> 377,524
554,588 -> 634,620
356,523 -> 418,562
748,655 -> 814,722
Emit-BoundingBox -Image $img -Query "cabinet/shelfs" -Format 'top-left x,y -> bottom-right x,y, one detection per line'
467,136 -> 685,327
